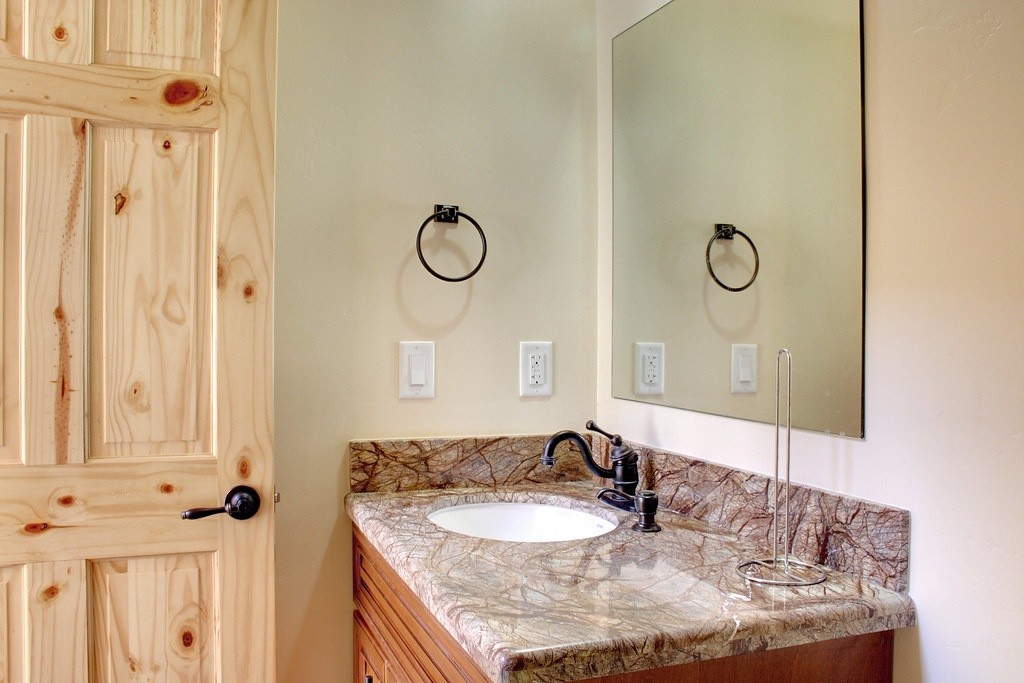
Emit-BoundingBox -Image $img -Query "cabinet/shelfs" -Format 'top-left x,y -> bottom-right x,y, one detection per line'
352,520 -> 894,683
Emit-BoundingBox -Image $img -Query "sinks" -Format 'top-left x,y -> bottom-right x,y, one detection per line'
423,492 -> 619,542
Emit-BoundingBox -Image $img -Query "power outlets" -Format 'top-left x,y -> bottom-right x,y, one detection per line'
635,342 -> 665,395
520,341 -> 553,396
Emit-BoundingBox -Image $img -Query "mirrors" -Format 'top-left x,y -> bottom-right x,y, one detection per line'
612,0 -> 865,439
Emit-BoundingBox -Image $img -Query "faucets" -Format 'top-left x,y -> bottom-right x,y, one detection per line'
540,419 -> 639,498
